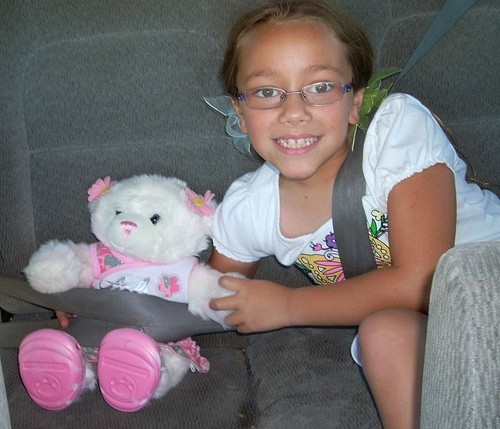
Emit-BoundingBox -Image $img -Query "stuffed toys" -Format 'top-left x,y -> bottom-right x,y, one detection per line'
18,173 -> 249,412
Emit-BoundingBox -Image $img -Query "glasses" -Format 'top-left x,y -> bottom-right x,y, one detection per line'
234,82 -> 353,110
